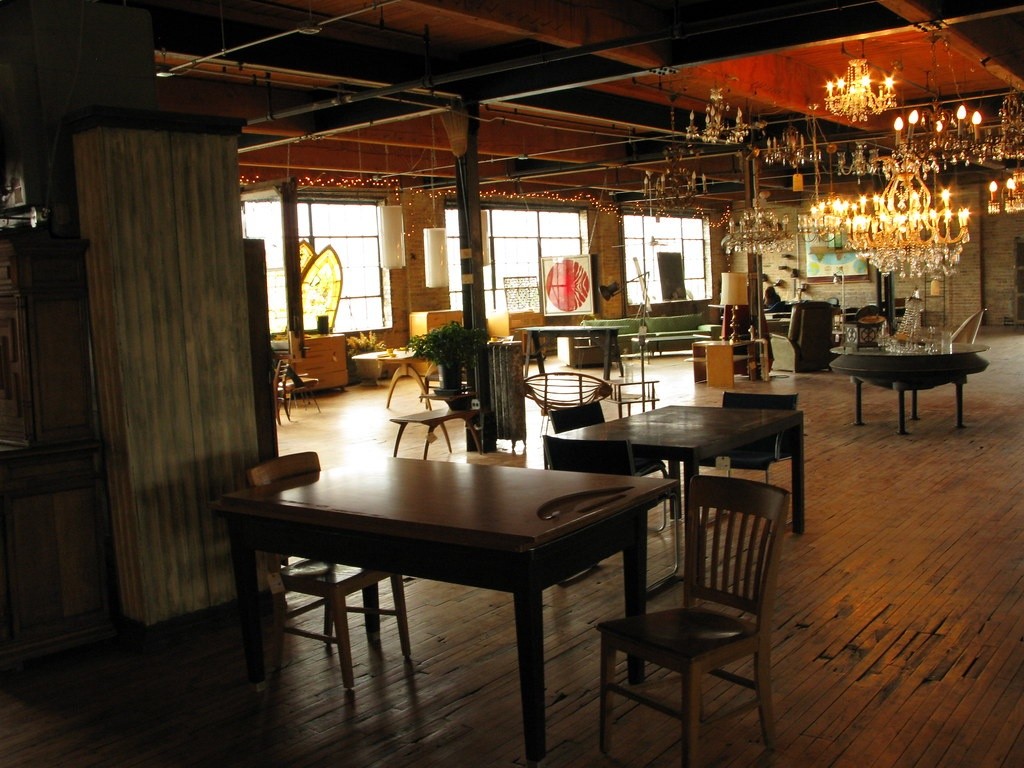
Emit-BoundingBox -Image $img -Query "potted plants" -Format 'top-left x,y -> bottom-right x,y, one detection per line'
403,321 -> 492,389
346,332 -> 388,389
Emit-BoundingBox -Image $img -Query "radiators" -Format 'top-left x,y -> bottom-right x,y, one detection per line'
485,340 -> 527,450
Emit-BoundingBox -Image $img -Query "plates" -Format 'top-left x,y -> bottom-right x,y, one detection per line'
380,354 -> 391,356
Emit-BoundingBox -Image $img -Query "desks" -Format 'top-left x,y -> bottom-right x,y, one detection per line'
516,326 -> 629,381
213,452 -> 678,768
542,406 -> 806,536
828,343 -> 990,436
350,349 -> 434,410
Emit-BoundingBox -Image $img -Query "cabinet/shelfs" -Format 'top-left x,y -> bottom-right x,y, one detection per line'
408,309 -> 463,376
0,228 -> 113,674
279,331 -> 348,394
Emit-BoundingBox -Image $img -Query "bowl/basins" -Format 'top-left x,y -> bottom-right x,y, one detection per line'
391,354 -> 396,358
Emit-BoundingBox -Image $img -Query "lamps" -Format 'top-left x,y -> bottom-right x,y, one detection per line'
375,141 -> 403,270
424,115 -> 450,289
720,271 -> 748,343
641,42 -> 1024,280
599,270 -> 650,361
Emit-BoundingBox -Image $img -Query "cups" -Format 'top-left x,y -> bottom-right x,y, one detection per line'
388,349 -> 393,355
942,332 -> 952,354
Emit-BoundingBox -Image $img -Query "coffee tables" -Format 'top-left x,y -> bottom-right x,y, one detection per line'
631,333 -> 709,357
685,339 -> 757,386
390,407 -> 482,461
603,380 -> 661,419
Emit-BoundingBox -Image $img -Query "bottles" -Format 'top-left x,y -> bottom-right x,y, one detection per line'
893,316 -> 902,330
878,320 -> 890,346
926,326 -> 937,353
285,327 -> 287,334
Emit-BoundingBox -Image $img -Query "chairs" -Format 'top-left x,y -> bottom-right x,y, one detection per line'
769,301 -> 838,374
524,367 -> 805,590
949,309 -> 987,343
271,341 -> 321,424
766,313 -> 791,331
596,474 -> 791,768
836,296 -> 908,349
247,453 -> 411,690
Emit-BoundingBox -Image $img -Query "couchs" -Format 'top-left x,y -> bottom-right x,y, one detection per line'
580,312 -> 723,356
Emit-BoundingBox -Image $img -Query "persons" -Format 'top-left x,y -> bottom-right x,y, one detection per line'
763,286 -> 781,309
